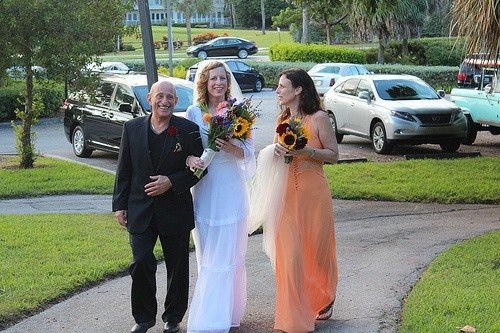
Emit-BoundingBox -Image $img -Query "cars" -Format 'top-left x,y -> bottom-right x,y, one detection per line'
79,61 -> 131,74
307,62 -> 374,96
186,59 -> 265,92
186,36 -> 258,60
322,73 -> 469,153
6,65 -> 47,80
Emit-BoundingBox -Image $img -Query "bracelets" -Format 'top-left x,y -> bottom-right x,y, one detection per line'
186,155 -> 194,167
310,148 -> 316,158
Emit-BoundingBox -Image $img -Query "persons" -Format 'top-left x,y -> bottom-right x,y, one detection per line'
184,59 -> 255,333
271,68 -> 339,333
111,79 -> 209,333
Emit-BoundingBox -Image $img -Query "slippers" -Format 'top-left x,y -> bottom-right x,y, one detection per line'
315,296 -> 336,320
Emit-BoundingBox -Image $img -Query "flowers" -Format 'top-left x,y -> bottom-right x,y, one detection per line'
275,111 -> 311,164
167,123 -> 180,141
187,94 -> 264,179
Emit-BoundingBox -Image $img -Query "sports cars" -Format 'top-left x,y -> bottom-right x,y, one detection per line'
451,67 -> 500,145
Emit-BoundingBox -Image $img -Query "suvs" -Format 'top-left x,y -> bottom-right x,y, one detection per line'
63,70 -> 195,158
457,53 -> 500,90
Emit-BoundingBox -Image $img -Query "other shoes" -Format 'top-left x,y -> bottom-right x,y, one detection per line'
163,320 -> 181,333
130,319 -> 156,333
